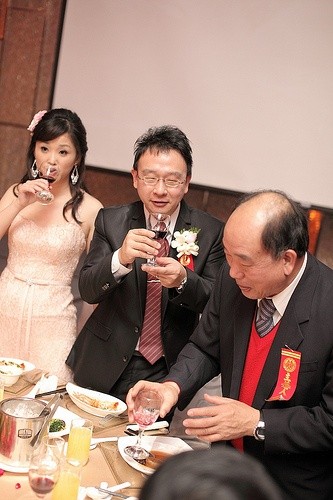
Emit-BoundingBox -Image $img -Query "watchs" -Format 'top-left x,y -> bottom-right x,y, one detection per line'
254,409 -> 266,441
176,278 -> 187,294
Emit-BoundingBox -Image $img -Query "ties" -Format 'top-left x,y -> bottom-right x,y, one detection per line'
255,298 -> 277,338
137,222 -> 171,366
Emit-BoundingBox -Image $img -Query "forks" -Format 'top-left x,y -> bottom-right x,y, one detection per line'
100,413 -> 128,426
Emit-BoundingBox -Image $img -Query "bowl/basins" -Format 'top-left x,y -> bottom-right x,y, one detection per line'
65,381 -> 128,417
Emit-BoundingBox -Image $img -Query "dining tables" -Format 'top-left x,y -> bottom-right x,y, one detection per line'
0,360 -> 201,500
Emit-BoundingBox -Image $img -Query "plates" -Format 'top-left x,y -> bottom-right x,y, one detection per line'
39,399 -> 85,438
0,447 -> 53,473
119,436 -> 194,476
0,357 -> 36,375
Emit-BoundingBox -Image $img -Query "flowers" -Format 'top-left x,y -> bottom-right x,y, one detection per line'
171,231 -> 199,258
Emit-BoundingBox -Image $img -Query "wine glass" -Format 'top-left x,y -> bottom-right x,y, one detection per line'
124,388 -> 164,459
34,160 -> 58,205
148,212 -> 170,266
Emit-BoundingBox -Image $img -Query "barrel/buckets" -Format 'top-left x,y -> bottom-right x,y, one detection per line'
0,397 -> 50,467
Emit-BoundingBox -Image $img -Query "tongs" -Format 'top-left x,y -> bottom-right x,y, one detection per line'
30,393 -> 63,450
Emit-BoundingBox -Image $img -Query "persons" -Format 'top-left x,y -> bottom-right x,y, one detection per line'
0,109 -> 104,387
65,124 -> 225,432
126,190 -> 333,500
139,444 -> 292,500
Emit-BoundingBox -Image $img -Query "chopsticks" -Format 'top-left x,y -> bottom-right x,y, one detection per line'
94,486 -> 129,499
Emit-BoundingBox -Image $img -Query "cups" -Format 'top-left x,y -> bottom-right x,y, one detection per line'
66,419 -> 93,466
52,466 -> 80,500
0,384 -> 4,401
28,454 -> 61,500
0,373 -> 19,387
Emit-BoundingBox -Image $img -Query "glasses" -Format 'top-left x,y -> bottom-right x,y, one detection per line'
136,174 -> 187,188
